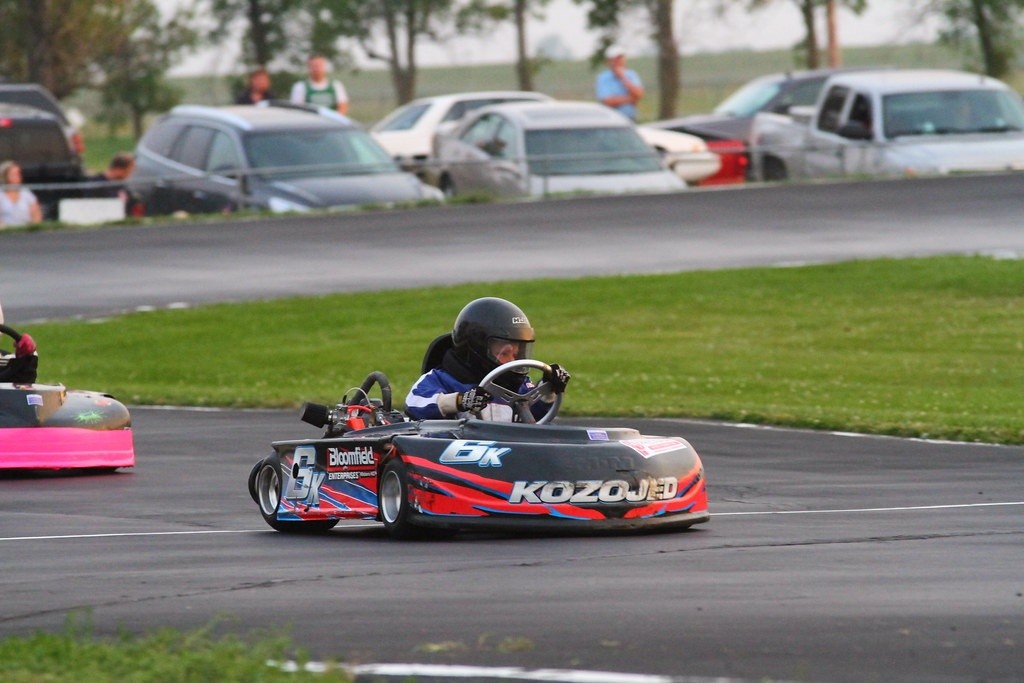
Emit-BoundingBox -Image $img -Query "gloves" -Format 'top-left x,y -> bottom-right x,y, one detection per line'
542,364 -> 571,395
13,334 -> 36,357
456,387 -> 494,415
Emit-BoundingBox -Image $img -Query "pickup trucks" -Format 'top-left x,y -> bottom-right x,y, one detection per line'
747,69 -> 1024,182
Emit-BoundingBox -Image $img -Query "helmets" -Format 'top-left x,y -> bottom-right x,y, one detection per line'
452,297 -> 536,400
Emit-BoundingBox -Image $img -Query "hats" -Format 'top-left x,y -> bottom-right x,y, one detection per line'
603,44 -> 625,59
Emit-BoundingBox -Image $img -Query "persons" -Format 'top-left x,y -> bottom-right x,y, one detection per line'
291,55 -> 348,115
404,297 -> 570,425
595,44 -> 644,123
237,71 -> 275,105
0,161 -> 41,227
81,153 -> 136,217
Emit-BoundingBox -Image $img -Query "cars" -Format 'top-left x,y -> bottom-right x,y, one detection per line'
425,101 -> 687,196
1,102 -> 83,217
642,70 -> 829,186
351,91 -> 554,164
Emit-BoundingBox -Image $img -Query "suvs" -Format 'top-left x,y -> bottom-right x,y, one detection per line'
124,99 -> 445,216
